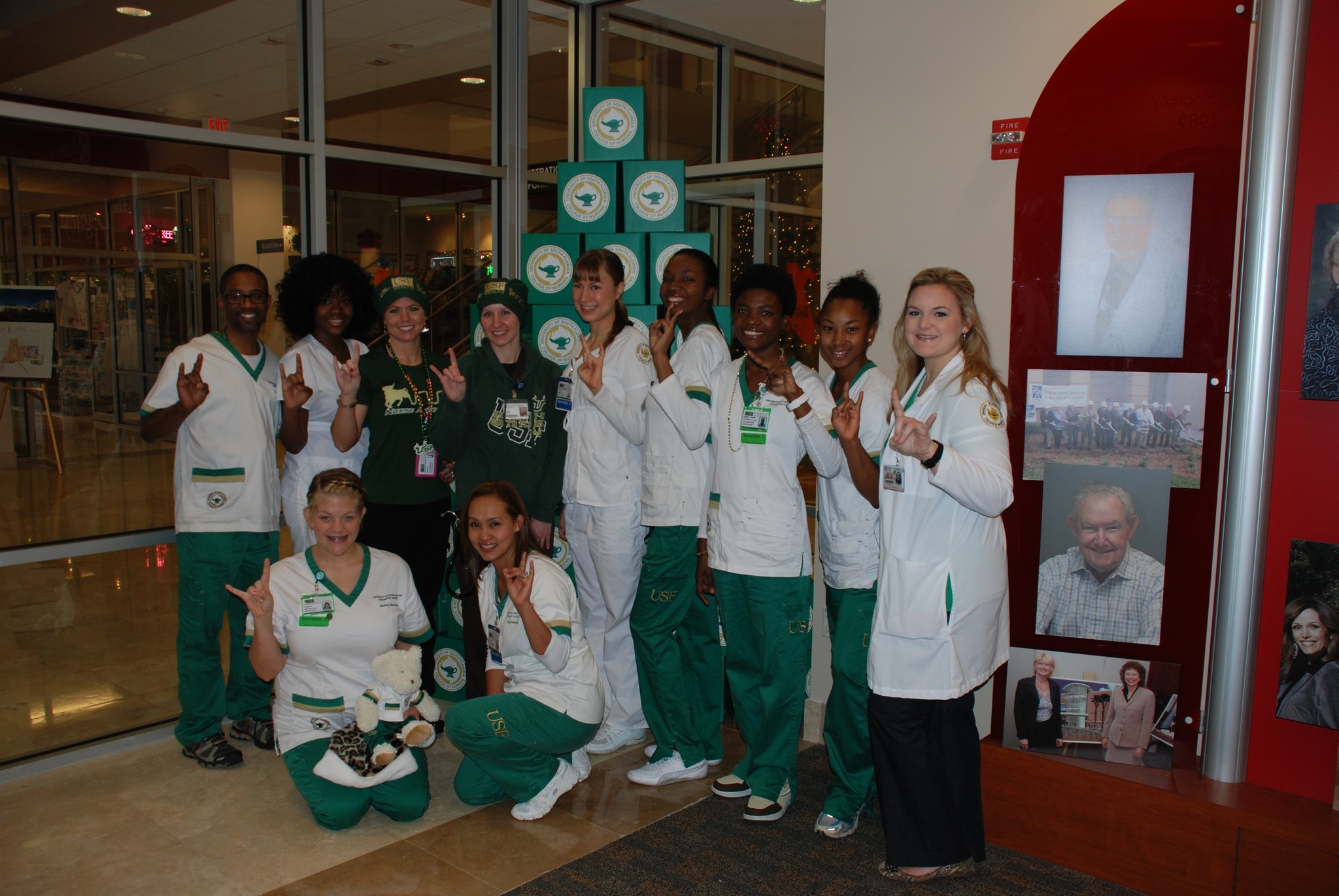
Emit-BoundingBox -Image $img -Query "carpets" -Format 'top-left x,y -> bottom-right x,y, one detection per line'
499,741 -> 1154,895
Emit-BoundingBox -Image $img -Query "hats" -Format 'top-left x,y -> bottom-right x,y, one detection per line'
477,278 -> 528,330
372,274 -> 430,320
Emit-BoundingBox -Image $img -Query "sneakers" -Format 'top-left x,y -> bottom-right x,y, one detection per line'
584,722 -> 646,755
626,749 -> 708,786
744,779 -> 792,821
814,802 -> 866,839
711,774 -> 752,797
511,757 -> 580,821
644,744 -> 723,765
182,731 -> 243,768
229,716 -> 275,750
572,746 -> 592,784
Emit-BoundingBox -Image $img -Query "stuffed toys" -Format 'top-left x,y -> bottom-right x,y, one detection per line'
355,645 -> 440,766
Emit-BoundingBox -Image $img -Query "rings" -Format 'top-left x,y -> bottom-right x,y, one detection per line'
451,471 -> 455,478
850,406 -> 856,410
522,572 -> 528,578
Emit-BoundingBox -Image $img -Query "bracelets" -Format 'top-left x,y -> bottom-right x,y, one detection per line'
787,391 -> 808,412
921,439 -> 944,469
336,395 -> 357,408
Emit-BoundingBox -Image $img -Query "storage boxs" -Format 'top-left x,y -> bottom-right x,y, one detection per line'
465,87 -> 735,360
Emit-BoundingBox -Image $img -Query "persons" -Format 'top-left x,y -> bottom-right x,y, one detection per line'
1278,596 -> 1339,730
866,266 -> 1014,884
436,277 -> 574,699
222,468 -> 439,832
331,274 -> 459,735
623,248 -> 729,789
445,480 -> 606,826
811,271 -> 898,838
1035,482 -> 1166,645
136,263 -> 290,769
556,248 -> 654,755
1300,230 -> 1339,401
1038,398 -> 1193,452
1011,653 -> 1155,767
269,253 -> 383,559
706,262 -> 845,822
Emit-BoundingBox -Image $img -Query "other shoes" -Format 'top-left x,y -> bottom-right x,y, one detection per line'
877,855 -> 975,882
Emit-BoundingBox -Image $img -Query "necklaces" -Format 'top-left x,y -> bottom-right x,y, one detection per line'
388,337 -> 434,442
727,346 -> 785,451
